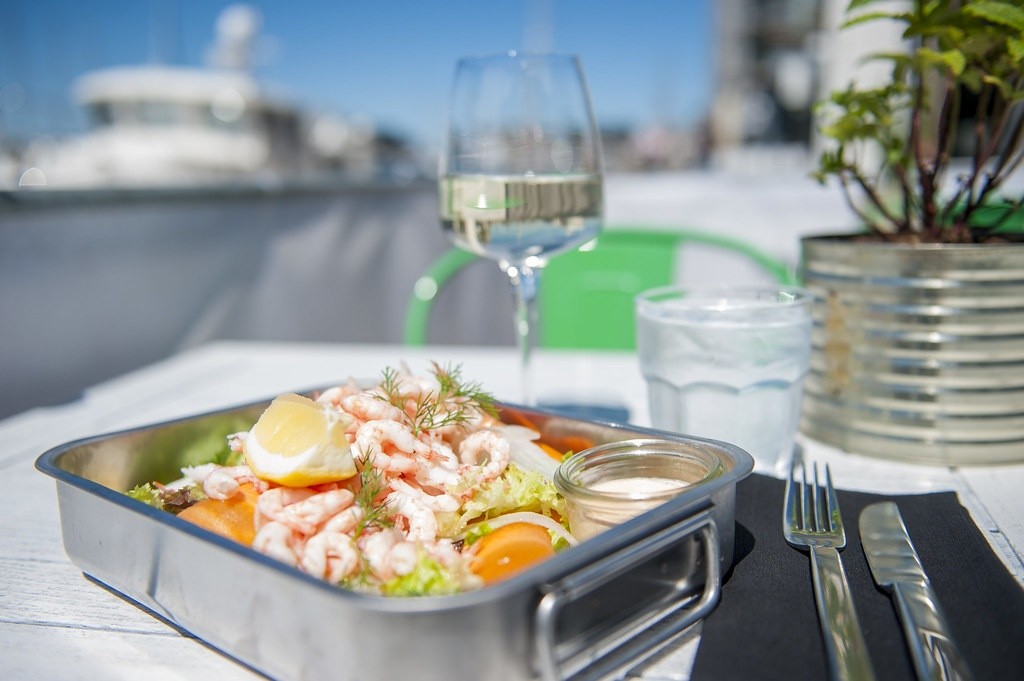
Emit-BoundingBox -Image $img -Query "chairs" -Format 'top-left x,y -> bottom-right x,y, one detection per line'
407,227 -> 790,351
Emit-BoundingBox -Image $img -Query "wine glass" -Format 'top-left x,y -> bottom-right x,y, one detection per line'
438,53 -> 607,411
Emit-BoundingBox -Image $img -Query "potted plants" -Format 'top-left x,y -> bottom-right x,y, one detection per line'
797,1 -> 1024,465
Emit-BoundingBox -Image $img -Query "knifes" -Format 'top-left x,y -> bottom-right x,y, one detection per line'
854,501 -> 973,681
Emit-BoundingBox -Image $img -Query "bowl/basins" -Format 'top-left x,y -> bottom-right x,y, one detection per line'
34,384 -> 757,681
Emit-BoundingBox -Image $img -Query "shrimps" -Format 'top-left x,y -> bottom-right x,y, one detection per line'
166,378 -> 510,592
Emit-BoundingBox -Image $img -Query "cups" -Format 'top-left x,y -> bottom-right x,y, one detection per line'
553,438 -> 724,556
635,287 -> 809,480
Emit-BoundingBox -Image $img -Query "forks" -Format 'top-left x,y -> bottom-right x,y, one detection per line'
783,460 -> 872,681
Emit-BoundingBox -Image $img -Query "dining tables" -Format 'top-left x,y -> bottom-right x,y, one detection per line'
1,341 -> 1024,681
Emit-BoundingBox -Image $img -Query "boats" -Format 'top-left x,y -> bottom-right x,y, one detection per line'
0,3 -> 406,187
